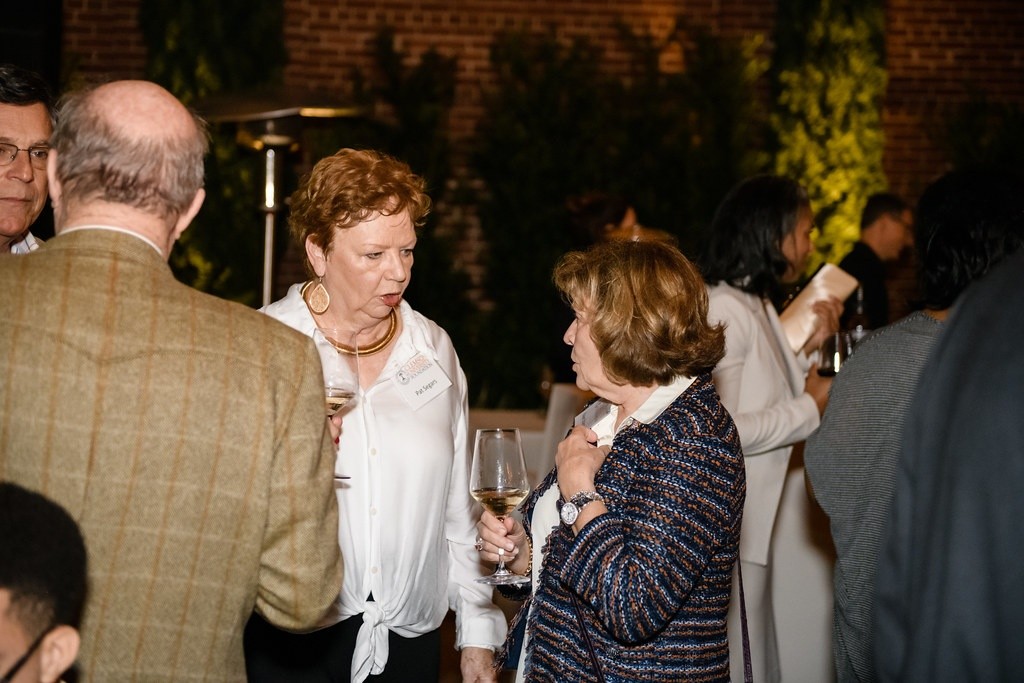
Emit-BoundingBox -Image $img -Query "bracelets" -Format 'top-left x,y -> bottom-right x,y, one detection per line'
505,533 -> 535,579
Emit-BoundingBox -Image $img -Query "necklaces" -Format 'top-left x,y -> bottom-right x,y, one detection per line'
302,279 -> 400,356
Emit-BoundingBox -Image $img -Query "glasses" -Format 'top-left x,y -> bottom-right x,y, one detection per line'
0,143 -> 49,170
896,216 -> 913,233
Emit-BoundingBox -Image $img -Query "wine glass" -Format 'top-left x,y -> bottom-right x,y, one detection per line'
469,428 -> 531,585
311,327 -> 359,480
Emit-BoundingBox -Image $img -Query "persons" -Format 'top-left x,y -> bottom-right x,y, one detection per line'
872,242 -> 1023,683
696,177 -> 846,683
0,67 -> 58,253
473,238 -> 747,683
605,191 -> 677,250
802,159 -> 1023,683
0,79 -> 346,682
839,192 -> 914,350
243,148 -> 510,682
1,479 -> 91,682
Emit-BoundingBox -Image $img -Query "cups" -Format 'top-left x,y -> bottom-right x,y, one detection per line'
817,333 -> 852,377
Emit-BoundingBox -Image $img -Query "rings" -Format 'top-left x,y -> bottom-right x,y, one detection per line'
475,537 -> 484,551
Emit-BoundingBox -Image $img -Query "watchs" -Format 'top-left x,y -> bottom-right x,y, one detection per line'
557,490 -> 606,528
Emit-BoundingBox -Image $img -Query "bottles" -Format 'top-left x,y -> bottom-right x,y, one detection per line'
845,284 -> 871,356
